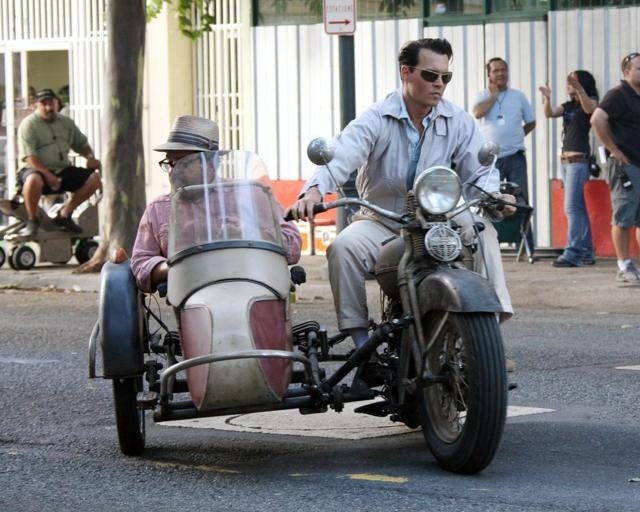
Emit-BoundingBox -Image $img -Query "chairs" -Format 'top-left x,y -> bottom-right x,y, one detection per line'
485,202 -> 536,265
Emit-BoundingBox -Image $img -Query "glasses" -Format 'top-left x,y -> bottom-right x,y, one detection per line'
409,66 -> 452,84
159,158 -> 198,172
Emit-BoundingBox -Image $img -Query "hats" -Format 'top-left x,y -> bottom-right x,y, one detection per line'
154,116 -> 219,152
34,89 -> 53,102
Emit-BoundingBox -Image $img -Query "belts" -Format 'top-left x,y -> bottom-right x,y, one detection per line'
561,156 -> 590,163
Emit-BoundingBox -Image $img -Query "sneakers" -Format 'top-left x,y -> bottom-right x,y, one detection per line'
553,256 -> 594,267
24,217 -> 38,237
617,263 -> 640,283
363,350 -> 380,378
56,212 -> 81,232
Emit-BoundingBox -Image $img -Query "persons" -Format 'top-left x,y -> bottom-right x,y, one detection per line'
589,52 -> 640,288
539,70 -> 599,268
283,38 -> 517,387
16,88 -> 101,240
472,57 -> 536,252
129,116 -> 301,293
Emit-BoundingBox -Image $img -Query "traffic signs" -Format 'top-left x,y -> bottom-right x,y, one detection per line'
324,1 -> 356,36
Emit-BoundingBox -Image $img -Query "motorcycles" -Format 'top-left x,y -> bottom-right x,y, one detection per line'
81,137 -> 528,481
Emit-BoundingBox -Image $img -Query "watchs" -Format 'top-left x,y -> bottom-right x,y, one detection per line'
86,153 -> 94,159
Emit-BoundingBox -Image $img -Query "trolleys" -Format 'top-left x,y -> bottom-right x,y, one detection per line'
1,162 -> 104,271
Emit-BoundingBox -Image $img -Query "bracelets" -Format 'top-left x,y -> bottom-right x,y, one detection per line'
609,145 -> 619,153
